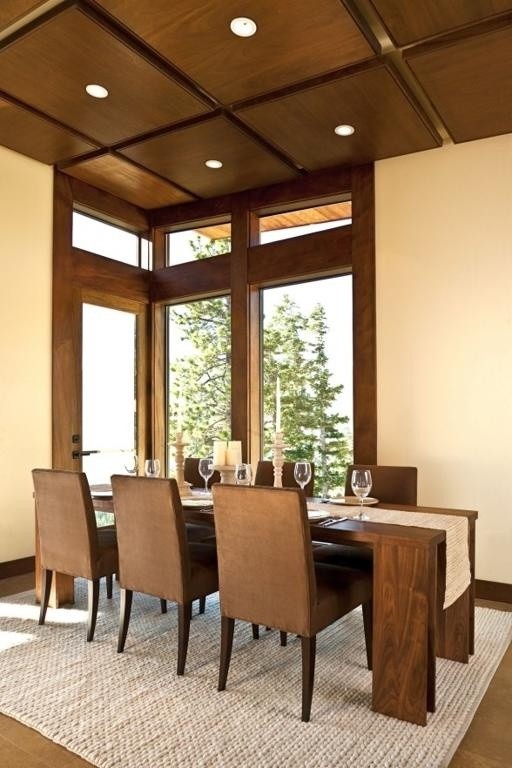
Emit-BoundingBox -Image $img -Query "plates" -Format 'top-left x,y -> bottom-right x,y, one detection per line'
306,511 -> 331,523
180,499 -> 213,510
329,495 -> 380,506
91,491 -> 114,498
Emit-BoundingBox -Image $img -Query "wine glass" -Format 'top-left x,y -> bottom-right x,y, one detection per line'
197,458 -> 214,497
350,469 -> 373,521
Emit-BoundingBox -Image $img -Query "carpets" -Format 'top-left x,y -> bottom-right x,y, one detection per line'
0,575 -> 512,767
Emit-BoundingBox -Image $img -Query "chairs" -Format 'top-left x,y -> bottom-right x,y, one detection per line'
297,465 -> 418,641
210,483 -> 374,724
161,456 -> 222,614
32,469 -> 169,641
110,473 -> 260,676
198,462 -> 316,638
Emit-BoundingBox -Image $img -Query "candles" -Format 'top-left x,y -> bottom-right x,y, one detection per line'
213,440 -> 242,466
275,376 -> 281,431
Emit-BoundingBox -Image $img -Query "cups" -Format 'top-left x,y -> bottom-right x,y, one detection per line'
293,462 -> 312,489
234,463 -> 253,485
123,454 -> 160,477
